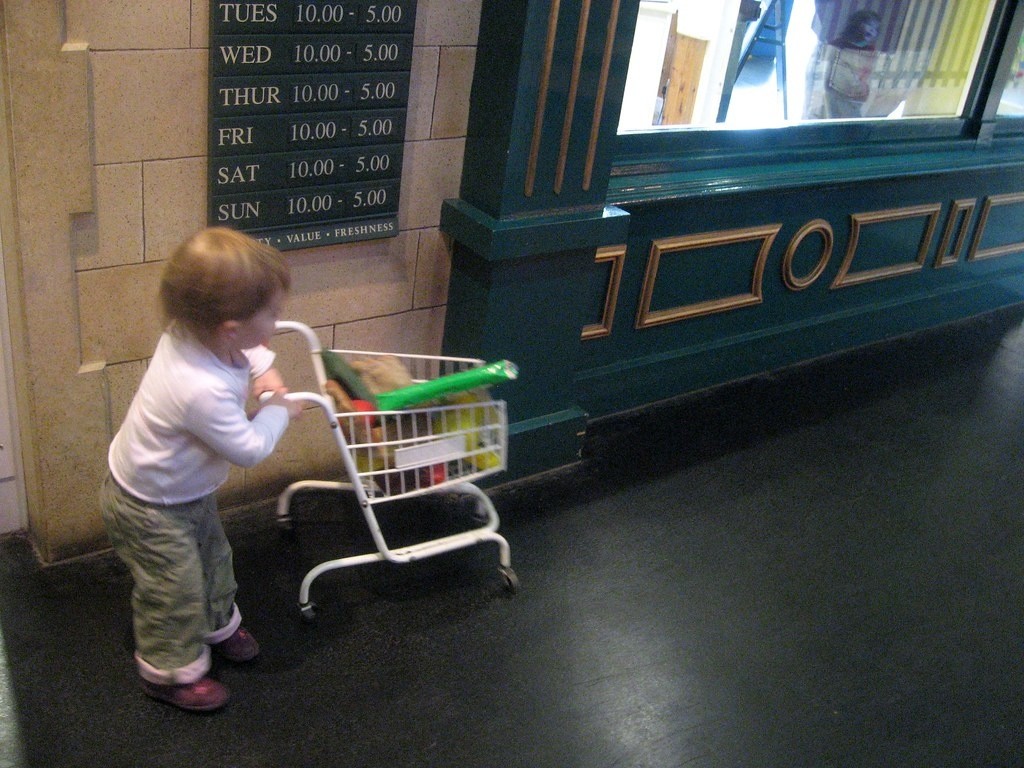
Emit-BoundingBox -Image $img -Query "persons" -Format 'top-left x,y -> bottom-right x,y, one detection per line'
801,0 -> 947,119
99,222 -> 311,711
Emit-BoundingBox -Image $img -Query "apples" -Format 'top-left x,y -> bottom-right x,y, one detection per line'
420,464 -> 445,485
338,399 -> 376,434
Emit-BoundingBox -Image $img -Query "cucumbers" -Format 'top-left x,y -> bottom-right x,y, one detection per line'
321,349 -> 373,401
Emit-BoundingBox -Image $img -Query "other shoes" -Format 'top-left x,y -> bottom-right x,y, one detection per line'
208,626 -> 260,661
139,677 -> 231,710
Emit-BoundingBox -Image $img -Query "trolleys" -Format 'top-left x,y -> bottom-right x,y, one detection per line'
257,318 -> 521,629
